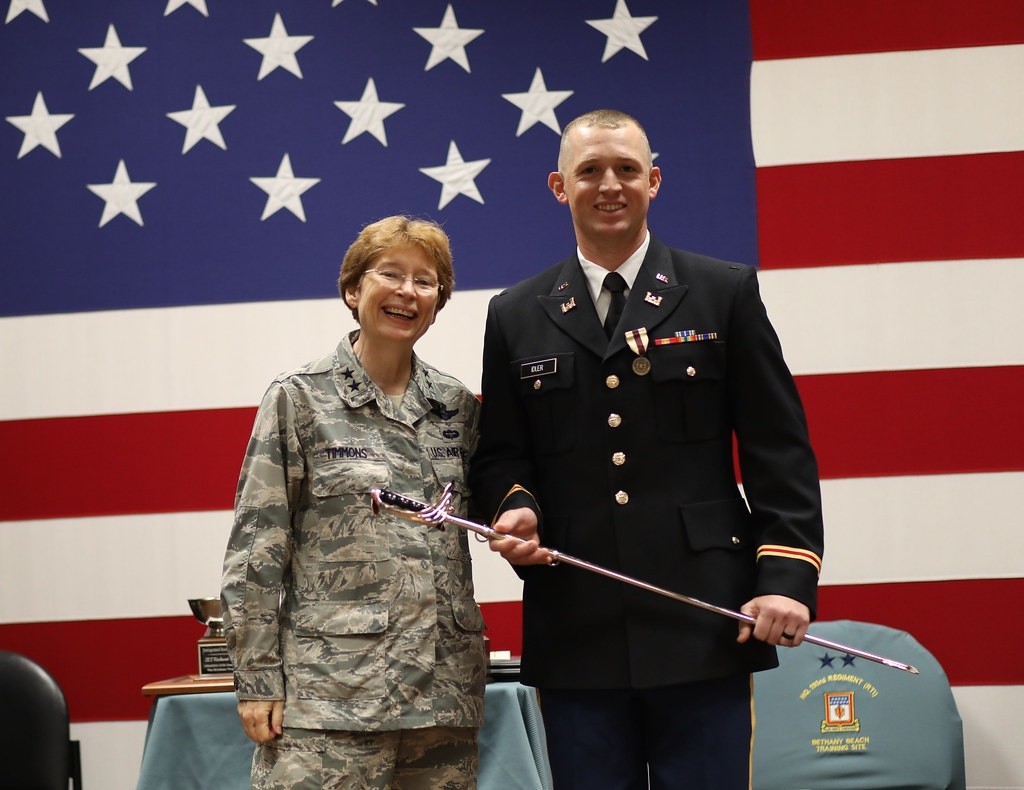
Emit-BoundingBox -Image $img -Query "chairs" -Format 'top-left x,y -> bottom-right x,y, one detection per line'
0,650 -> 82,790
749,621 -> 968,790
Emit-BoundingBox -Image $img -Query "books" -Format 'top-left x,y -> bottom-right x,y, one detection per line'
142,675 -> 235,694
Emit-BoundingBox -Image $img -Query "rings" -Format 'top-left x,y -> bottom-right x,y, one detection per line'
781,631 -> 795,641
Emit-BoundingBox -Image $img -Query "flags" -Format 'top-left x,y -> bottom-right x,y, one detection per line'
0,0 -> 1024,790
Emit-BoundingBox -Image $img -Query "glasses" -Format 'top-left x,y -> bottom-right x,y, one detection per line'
362,268 -> 443,291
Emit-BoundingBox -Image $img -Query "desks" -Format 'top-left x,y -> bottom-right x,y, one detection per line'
137,676 -> 554,790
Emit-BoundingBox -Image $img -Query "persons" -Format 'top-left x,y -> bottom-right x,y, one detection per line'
469,109 -> 824,790
219,214 -> 485,790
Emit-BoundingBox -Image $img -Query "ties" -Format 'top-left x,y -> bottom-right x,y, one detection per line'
603,272 -> 629,342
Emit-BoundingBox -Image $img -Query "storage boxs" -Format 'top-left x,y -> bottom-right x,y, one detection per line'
199,638 -> 235,678
